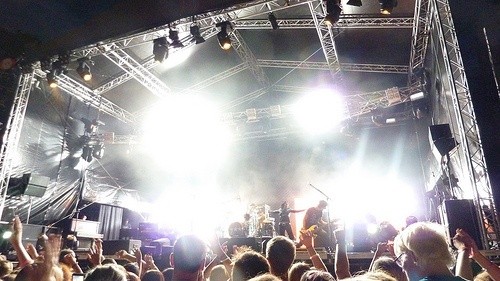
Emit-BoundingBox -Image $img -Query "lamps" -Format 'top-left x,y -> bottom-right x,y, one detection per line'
77,58 -> 94,81
46,69 -> 59,89
215,21 -> 231,51
153,37 -> 170,65
379,0 -> 397,15
324,0 -> 342,27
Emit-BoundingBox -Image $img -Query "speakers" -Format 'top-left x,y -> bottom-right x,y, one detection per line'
19,173 -> 50,198
219,237 -> 256,257
442,199 -> 484,250
428,124 -> 456,164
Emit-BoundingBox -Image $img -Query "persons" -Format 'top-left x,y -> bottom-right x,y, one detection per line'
0,199 -> 500,281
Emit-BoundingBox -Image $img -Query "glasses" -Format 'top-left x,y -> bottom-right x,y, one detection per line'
395,253 -> 404,267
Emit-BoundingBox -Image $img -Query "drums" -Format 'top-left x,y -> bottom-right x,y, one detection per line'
228,221 -> 248,238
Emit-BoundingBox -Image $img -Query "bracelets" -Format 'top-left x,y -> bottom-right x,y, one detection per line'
335,240 -> 347,244
458,246 -> 471,253
306,244 -> 312,249
482,261 -> 493,270
310,253 -> 319,260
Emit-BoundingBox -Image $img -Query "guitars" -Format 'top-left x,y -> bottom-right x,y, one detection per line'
299,218 -> 341,241
